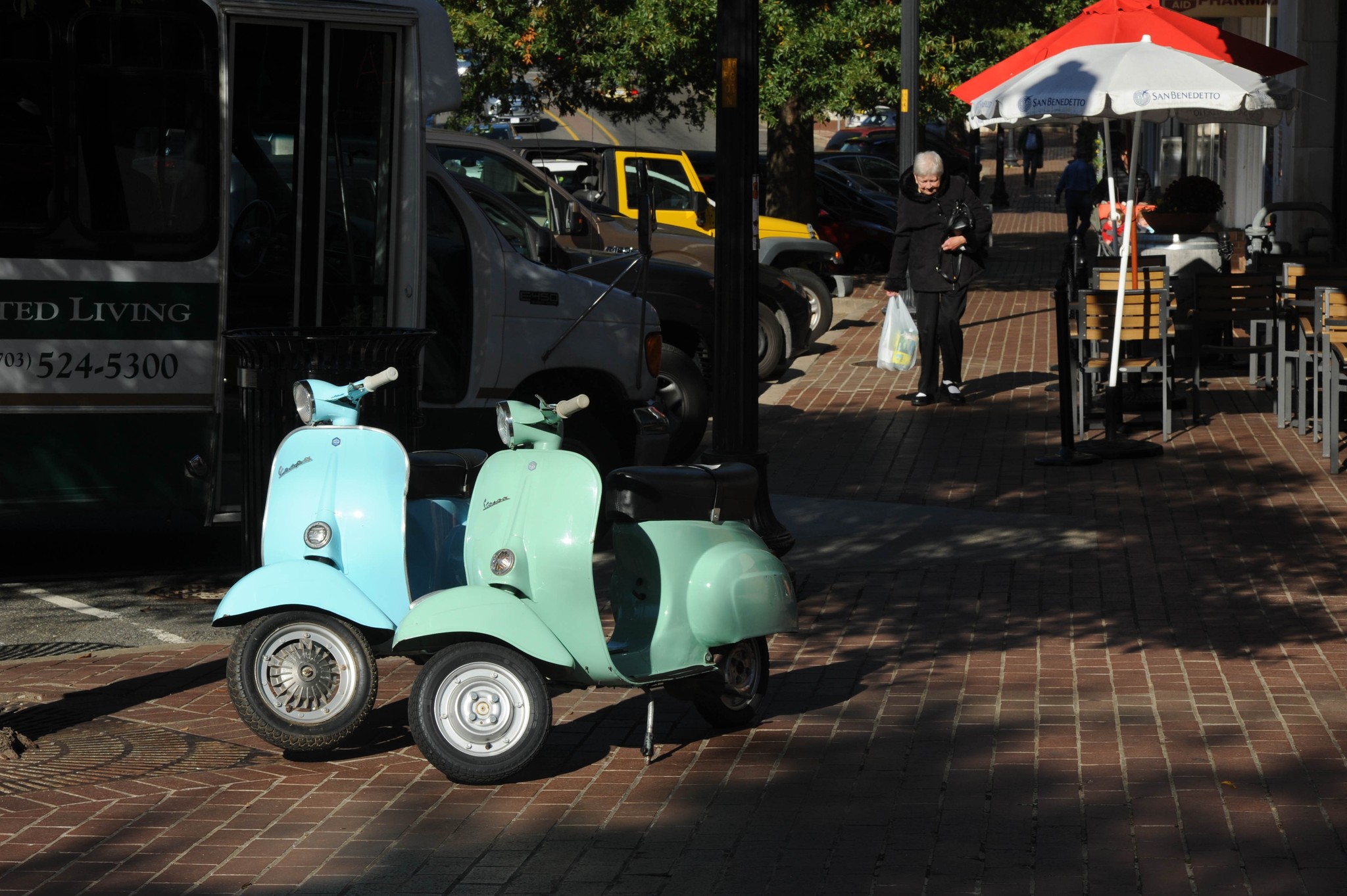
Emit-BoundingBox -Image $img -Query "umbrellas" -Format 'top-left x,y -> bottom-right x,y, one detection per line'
948,0 -> 1309,388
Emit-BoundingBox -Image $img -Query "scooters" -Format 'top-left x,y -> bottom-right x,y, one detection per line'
391,391 -> 797,786
211,365 -> 491,750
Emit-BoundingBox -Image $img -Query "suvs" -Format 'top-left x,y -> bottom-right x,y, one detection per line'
424,106 -> 972,387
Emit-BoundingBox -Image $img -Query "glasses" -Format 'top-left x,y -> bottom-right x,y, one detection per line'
1124,153 -> 1131,160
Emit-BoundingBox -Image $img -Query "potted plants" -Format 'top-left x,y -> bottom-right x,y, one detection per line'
1139,174 -> 1226,235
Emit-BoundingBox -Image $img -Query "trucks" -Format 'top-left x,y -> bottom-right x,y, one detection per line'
2,0 -> 684,543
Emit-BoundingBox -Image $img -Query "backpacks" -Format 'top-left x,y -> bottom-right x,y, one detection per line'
1026,129 -> 1039,152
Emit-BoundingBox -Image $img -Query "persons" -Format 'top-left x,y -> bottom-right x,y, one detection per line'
1054,149 -> 1098,247
885,152 -> 993,407
1103,145 -> 1149,206
1018,124 -> 1043,186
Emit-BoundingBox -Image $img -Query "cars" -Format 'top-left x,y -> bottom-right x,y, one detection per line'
449,173 -> 712,362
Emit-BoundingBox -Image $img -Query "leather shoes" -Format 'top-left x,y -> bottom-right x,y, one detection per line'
941,380 -> 966,404
912,391 -> 941,405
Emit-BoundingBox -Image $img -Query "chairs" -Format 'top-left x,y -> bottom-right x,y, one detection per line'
1077,254 -> 1347,475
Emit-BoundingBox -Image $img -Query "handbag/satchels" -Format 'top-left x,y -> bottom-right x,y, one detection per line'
876,294 -> 919,375
935,202 -> 989,283
